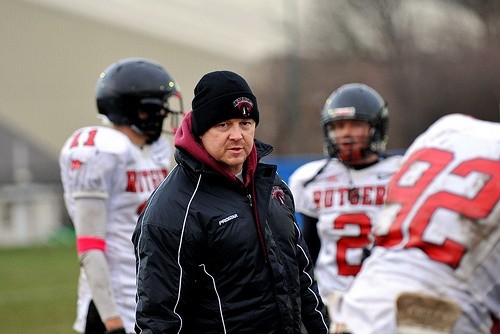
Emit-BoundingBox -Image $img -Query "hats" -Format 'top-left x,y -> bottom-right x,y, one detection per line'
191,70 -> 259,138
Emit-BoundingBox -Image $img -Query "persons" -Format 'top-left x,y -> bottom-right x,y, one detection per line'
329,113 -> 500,334
287,82 -> 404,328
57,56 -> 172,334
130,70 -> 330,334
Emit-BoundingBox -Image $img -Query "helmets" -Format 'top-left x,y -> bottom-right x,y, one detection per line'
95,59 -> 177,114
320,82 -> 391,157
